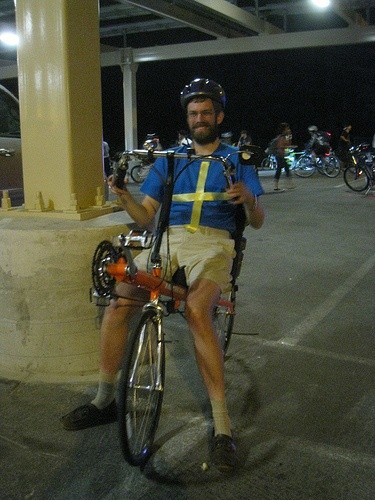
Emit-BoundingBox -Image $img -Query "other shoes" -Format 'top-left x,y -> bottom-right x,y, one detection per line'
60,397 -> 119,430
274,188 -> 284,192
211,427 -> 237,472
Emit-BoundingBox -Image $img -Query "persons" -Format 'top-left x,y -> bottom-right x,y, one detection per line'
59,79 -> 265,473
337,123 -> 352,163
273,123 -> 292,190
237,130 -> 251,147
103,141 -> 113,178
178,130 -> 192,146
308,126 -> 330,164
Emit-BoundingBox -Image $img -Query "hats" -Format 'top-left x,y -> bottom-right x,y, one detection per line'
308,125 -> 318,131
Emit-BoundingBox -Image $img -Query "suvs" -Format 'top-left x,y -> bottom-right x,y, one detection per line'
0,81 -> 23,201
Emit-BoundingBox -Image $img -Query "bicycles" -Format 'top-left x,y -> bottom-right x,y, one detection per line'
123,149 -> 174,183
344,142 -> 375,194
262,150 -> 296,170
289,150 -> 339,179
78,140 -> 249,473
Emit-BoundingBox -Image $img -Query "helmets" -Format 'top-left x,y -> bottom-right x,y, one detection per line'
181,78 -> 227,109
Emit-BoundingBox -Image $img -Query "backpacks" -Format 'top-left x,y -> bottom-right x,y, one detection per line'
315,130 -> 332,142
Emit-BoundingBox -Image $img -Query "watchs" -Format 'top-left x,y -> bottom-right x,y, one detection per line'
252,194 -> 256,209
